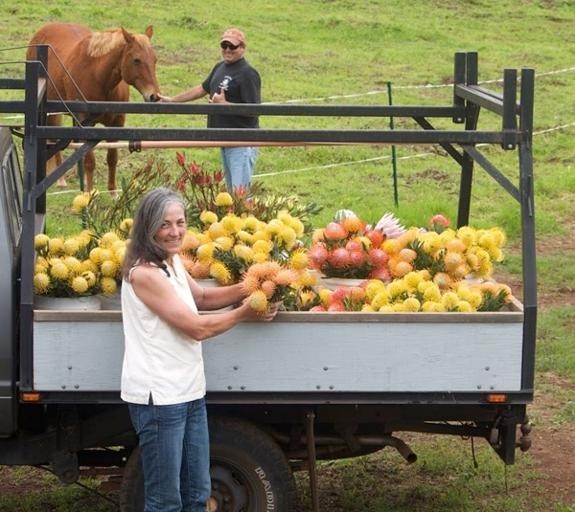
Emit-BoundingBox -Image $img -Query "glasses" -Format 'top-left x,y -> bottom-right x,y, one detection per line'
221,43 -> 240,50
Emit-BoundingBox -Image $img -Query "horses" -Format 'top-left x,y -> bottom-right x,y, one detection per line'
26,23 -> 161,201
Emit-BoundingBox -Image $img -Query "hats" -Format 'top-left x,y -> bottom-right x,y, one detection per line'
221,29 -> 247,47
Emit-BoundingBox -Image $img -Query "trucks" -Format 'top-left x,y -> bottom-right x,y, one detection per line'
0,45 -> 536,512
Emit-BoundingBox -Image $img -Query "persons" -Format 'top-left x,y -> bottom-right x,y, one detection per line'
156,28 -> 261,200
120,186 -> 283,512
